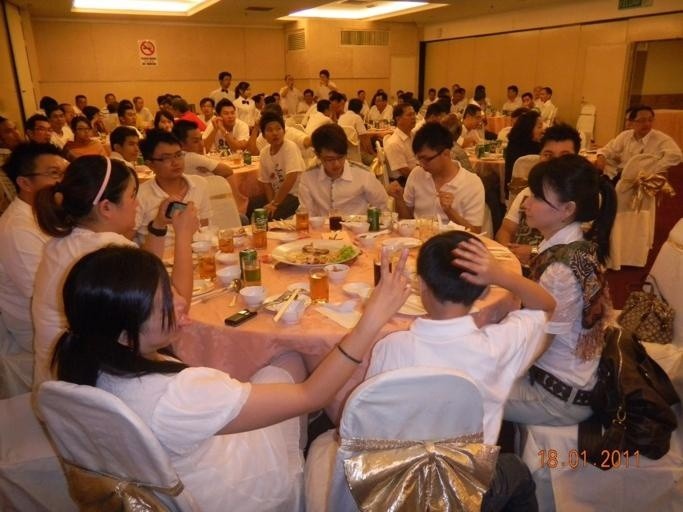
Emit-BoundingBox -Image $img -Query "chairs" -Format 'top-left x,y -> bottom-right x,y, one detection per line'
523,352 -> 683,512
375,140 -> 391,187
305,369 -> 501,512
37,379 -> 202,511
604,218 -> 683,378
506,154 -> 540,210
598,154 -> 666,271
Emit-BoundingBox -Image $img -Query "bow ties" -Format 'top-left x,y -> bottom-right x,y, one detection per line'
241,99 -> 250,104
221,89 -> 228,93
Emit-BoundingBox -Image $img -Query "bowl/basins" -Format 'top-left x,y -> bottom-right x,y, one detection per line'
357,234 -> 377,246
358,288 -> 372,304
398,223 -> 416,236
325,264 -> 350,282
309,216 -> 323,225
189,240 -> 210,253
351,221 -> 369,235
276,301 -> 304,323
218,266 -> 242,283
240,287 -> 265,307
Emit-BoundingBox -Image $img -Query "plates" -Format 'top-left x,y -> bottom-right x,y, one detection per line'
218,254 -> 238,263
340,282 -> 371,295
272,238 -> 359,267
261,292 -> 313,311
398,293 -> 479,316
287,281 -> 310,294
339,212 -> 398,228
383,237 -> 423,249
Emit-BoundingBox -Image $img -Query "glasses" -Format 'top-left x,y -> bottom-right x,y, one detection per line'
318,153 -> 346,163
416,148 -> 444,162
22,166 -> 64,179
33,126 -> 54,134
153,152 -> 186,162
633,116 -> 656,123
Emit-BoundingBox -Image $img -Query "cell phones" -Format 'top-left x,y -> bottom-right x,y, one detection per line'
225,307 -> 257,325
165,201 -> 188,219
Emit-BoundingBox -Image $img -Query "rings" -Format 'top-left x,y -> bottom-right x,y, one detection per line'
446,195 -> 448,198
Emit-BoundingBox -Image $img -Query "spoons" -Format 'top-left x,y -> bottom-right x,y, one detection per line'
319,301 -> 356,313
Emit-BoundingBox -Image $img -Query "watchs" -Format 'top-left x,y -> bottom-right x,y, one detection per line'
531,244 -> 538,259
147,221 -> 168,236
271,199 -> 280,207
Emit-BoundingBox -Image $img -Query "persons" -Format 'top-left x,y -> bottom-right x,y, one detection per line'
503,112 -> 543,177
371,88 -> 383,107
24,114 -> 52,145
176,98 -> 207,131
328,90 -> 347,101
119,100 -> 133,105
503,153 -> 618,426
533,87 -> 541,98
170,119 -> 234,178
49,245 -> 411,512
153,110 -> 175,132
80,106 -> 105,134
520,92 -> 539,116
111,126 -> 139,169
365,93 -> 393,126
258,111 -> 302,221
363,230 -> 557,446
534,87 -> 559,128
132,96 -> 154,122
298,124 -> 388,216
32,153 -> 199,412
317,69 -> 337,102
49,106 -> 74,148
590,107 -> 633,154
209,72 -> 235,103
40,96 -> 57,116
273,93 -> 280,104
337,98 -> 366,134
0,115 -> 25,214
122,128 -> 212,247
357,90 -> 369,119
307,99 -> 335,136
59,103 -> 76,122
156,94 -> 180,110
329,93 -> 366,114
203,97 -> 249,154
264,96 -> 276,107
386,121 -> 485,234
396,83 -> 492,120
75,94 -> 87,110
597,105 -> 682,176
498,119 -> 581,276
251,93 -> 265,109
247,103 -> 312,155
280,75 -> 303,116
0,141 -> 71,357
102,94 -> 116,110
296,88 -> 316,114
62,116 -> 109,161
107,106 -> 145,139
301,100 -> 318,126
502,86 -> 521,114
107,101 -> 120,116
233,81 -> 256,137
199,97 -> 215,128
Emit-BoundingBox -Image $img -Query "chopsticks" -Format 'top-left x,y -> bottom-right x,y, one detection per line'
273,286 -> 301,322
191,288 -> 229,307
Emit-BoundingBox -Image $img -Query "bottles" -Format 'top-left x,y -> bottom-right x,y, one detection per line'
244,151 -> 251,165
367,207 -> 380,231
238,248 -> 257,278
253,208 -> 268,231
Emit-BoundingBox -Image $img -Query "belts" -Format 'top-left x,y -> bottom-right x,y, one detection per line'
528,364 -> 592,407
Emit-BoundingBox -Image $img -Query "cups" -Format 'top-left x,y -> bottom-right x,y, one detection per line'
328,208 -> 343,232
309,268 -> 329,303
217,230 -> 233,253
251,219 -> 266,249
419,215 -> 433,237
242,258 -> 262,286
197,250 -> 216,282
295,209 -> 309,233
373,247 -> 392,285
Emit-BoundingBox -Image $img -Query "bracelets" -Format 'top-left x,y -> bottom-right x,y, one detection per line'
337,344 -> 362,364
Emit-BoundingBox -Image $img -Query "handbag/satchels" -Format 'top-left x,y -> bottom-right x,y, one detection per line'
616,272 -> 676,345
576,324 -> 681,471
